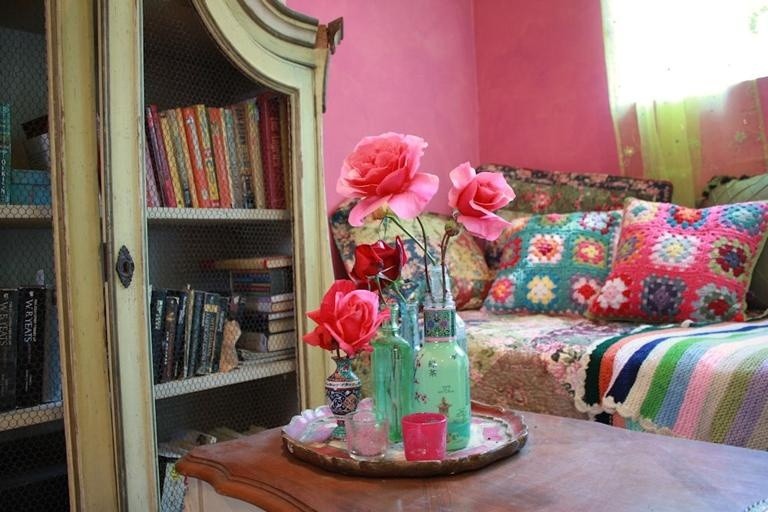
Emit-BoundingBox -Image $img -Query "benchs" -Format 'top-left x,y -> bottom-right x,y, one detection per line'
377,310 -> 768,454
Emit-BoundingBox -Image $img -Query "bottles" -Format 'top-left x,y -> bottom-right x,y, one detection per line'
368,266 -> 470,455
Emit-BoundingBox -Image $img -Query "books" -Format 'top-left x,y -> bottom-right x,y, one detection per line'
160,421 -> 275,511
144,98 -> 289,210
0,101 -> 12,202
0,283 -> 65,410
153,255 -> 296,381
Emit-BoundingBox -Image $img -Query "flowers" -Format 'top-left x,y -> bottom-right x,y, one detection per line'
302,277 -> 390,358
350,235 -> 425,346
334,130 -> 516,304
360,198 -> 445,288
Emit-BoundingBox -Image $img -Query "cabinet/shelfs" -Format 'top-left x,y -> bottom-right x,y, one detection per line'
0,0 -> 347,512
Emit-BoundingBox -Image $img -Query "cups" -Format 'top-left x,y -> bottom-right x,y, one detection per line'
401,412 -> 447,460
343,409 -> 388,462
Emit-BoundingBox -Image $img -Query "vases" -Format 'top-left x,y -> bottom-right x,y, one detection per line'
326,261 -> 472,455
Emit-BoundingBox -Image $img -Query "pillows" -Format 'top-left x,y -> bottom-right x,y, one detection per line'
697,170 -> 768,318
582,195 -> 768,324
329,203 -> 492,313
475,164 -> 672,213
480,207 -> 624,323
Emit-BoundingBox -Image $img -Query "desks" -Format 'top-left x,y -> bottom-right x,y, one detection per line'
174,394 -> 768,512
